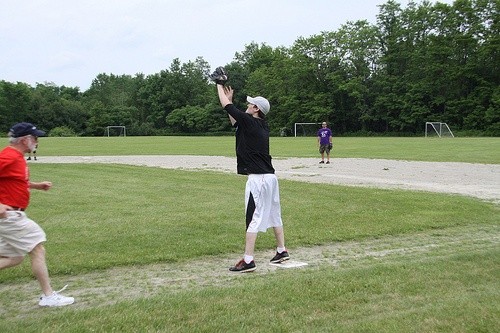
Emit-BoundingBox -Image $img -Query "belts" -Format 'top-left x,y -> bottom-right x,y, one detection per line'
6,207 -> 24,211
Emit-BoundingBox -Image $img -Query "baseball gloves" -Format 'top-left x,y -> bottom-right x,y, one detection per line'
208,66 -> 229,85
327,142 -> 333,151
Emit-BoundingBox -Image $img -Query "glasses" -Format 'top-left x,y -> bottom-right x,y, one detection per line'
32,135 -> 37,140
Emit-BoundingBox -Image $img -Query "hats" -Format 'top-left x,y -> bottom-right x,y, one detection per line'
247,96 -> 271,115
322,122 -> 327,124
10,122 -> 45,137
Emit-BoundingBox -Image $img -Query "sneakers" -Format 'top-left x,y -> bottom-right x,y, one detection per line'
39,284 -> 74,307
229,257 -> 257,273
270,248 -> 290,263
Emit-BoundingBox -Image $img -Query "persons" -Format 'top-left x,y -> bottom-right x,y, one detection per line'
317,122 -> 333,164
0,122 -> 75,307
210,66 -> 291,274
26,136 -> 39,160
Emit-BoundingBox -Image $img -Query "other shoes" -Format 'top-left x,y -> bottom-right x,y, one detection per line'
319,161 -> 324,164
27,156 -> 31,160
34,156 -> 37,160
326,161 -> 330,164
